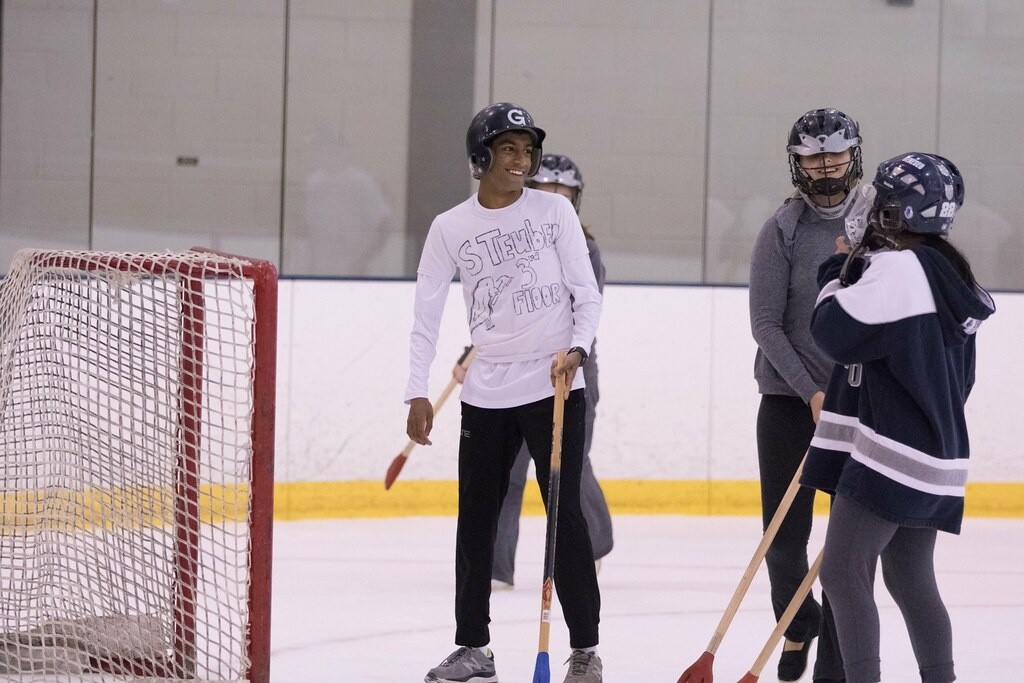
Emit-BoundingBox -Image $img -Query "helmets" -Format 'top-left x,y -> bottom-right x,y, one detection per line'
866,151 -> 965,247
466,102 -> 546,180
786,108 -> 863,220
524,153 -> 584,213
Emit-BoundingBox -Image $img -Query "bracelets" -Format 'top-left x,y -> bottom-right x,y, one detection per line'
567,346 -> 588,367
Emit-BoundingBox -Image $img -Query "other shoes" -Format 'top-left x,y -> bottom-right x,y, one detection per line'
595,559 -> 601,573
491,580 -> 513,590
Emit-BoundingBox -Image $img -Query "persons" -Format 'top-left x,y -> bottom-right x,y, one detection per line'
403,101 -> 602,683
800,151 -> 996,683
750,108 -> 873,683
454,154 -> 613,589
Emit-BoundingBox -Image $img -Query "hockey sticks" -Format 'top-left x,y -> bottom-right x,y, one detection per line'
676,448 -> 811,683
384,345 -> 475,490
735,544 -> 824,682
533,351 -> 567,683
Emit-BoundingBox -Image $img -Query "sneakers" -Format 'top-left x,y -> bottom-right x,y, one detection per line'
777,638 -> 814,682
424,647 -> 499,683
562,649 -> 602,683
